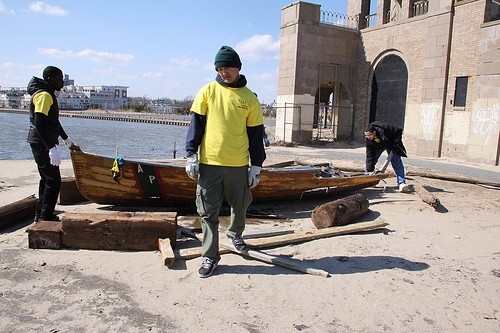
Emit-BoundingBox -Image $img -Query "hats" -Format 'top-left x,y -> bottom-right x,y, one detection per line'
214,46 -> 241,71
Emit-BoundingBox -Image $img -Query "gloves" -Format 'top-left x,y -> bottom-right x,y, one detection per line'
64,136 -> 77,148
185,154 -> 199,181
48,147 -> 62,167
248,166 -> 262,188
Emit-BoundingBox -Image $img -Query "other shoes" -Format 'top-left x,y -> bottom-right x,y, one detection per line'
33,215 -> 59,222
398,184 -> 408,193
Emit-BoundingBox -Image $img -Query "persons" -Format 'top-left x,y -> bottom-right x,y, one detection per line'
26,66 -> 75,222
185,46 -> 266,277
364,121 -> 409,191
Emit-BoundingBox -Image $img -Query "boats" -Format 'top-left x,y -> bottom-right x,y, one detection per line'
67,144 -> 395,207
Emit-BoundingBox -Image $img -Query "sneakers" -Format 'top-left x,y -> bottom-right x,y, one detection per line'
232,236 -> 248,254
198,255 -> 218,278
